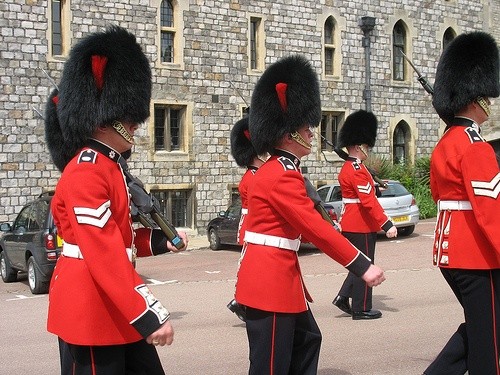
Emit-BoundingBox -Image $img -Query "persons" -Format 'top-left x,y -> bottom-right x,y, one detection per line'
227,115 -> 271,322
46,25 -> 188,375
420,31 -> 500,375
235,55 -> 385,375
332,109 -> 397,320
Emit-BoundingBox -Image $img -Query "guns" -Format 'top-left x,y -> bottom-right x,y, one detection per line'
313,131 -> 390,189
37,64 -> 186,251
229,80 -> 341,234
399,50 -> 434,95
29,103 -> 159,229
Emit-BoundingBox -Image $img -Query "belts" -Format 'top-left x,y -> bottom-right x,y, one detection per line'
342,197 -> 362,203
437,201 -> 473,210
243,231 -> 301,251
62,241 -> 138,263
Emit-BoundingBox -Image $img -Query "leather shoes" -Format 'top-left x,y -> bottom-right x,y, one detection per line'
226,299 -> 246,322
353,308 -> 382,320
332,295 -> 352,314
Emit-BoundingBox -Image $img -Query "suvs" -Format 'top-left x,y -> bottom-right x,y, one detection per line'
0,188 -> 64,294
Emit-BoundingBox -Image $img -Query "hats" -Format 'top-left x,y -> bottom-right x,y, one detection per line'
56,24 -> 153,148
431,32 -> 500,124
44,87 -> 83,173
249,53 -> 321,154
230,118 -> 258,167
338,110 -> 378,148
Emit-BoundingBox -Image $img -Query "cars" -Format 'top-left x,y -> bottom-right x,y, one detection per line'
314,179 -> 420,238
206,194 -> 342,252
